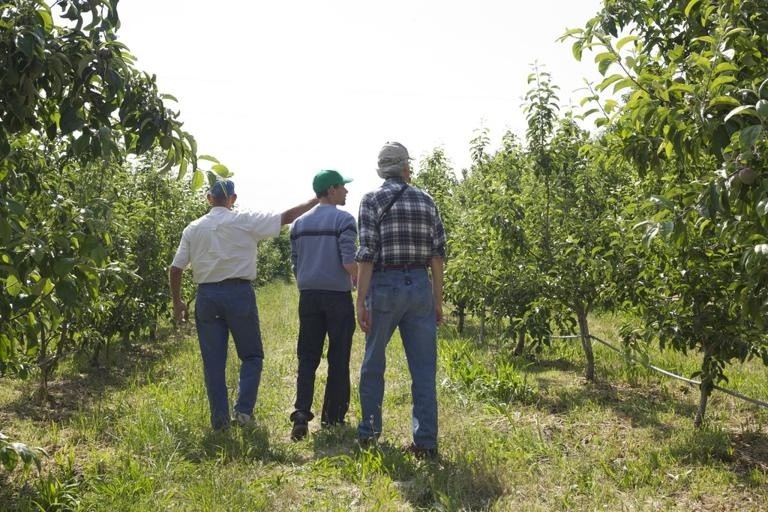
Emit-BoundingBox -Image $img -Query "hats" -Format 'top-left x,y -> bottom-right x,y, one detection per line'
210,180 -> 234,195
313,169 -> 353,192
378,141 -> 416,167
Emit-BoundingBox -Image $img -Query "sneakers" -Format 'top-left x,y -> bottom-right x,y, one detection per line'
403,444 -> 438,454
290,419 -> 308,442
230,408 -> 256,426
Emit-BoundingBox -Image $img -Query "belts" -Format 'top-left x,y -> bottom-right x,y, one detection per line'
217,279 -> 249,284
384,265 -> 425,268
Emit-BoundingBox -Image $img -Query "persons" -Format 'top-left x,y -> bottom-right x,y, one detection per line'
290,169 -> 358,443
356,142 -> 446,459
171,179 -> 318,430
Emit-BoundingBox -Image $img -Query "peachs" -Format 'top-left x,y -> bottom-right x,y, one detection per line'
729,173 -> 741,188
738,168 -> 756,186
